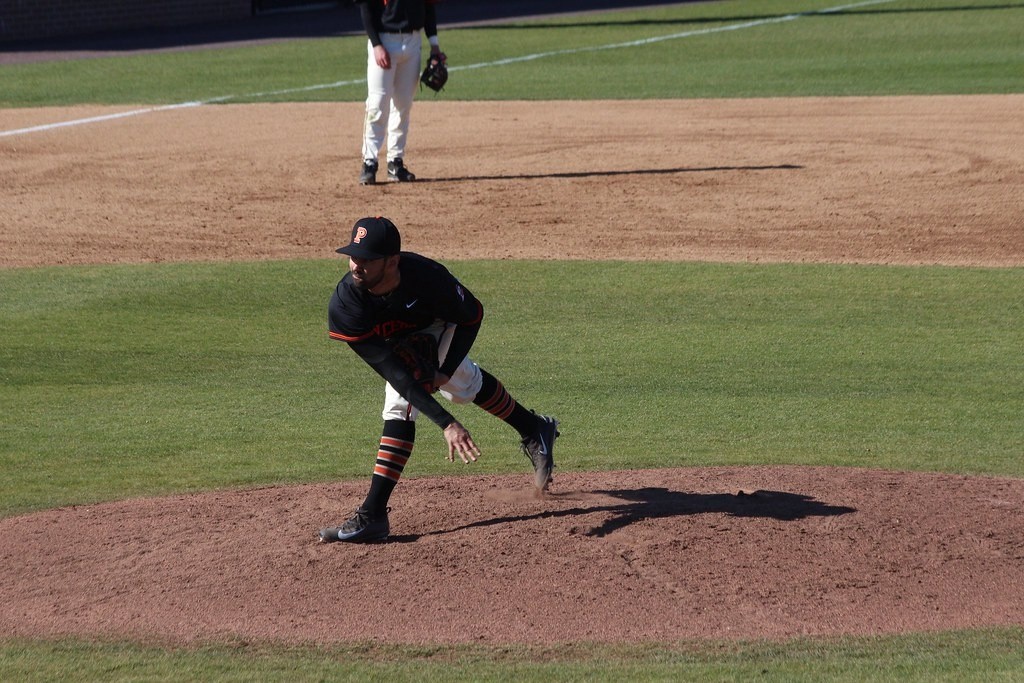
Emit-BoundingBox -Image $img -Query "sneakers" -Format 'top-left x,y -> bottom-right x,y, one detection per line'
319,506 -> 392,543
386,158 -> 415,182
359,159 -> 378,185
518,408 -> 561,492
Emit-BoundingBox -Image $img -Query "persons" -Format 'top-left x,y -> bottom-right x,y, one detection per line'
319,214 -> 560,544
359,0 -> 444,184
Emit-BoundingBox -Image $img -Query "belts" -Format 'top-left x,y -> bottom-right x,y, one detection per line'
378,22 -> 422,34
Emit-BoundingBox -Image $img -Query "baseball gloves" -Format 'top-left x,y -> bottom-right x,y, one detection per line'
421,56 -> 449,89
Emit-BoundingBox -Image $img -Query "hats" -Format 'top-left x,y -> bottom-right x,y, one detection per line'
334,215 -> 401,260
